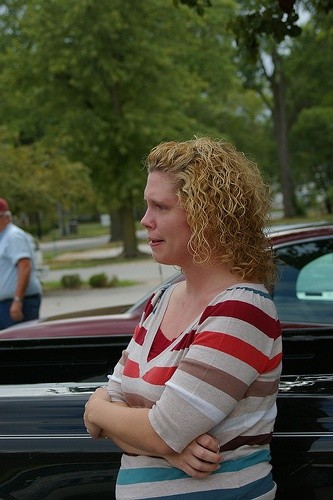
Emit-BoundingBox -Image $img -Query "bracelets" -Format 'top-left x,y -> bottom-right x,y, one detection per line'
14,296 -> 23,302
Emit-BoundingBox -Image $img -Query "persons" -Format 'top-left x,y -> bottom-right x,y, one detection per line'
0,197 -> 42,331
84,136 -> 282,500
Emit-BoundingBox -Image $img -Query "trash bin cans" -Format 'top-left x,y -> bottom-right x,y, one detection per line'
65,220 -> 79,235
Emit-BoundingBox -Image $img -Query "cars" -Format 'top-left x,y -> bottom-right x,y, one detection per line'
0,222 -> 333,500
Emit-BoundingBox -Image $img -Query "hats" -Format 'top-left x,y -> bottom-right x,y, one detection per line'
0,198 -> 9,211
